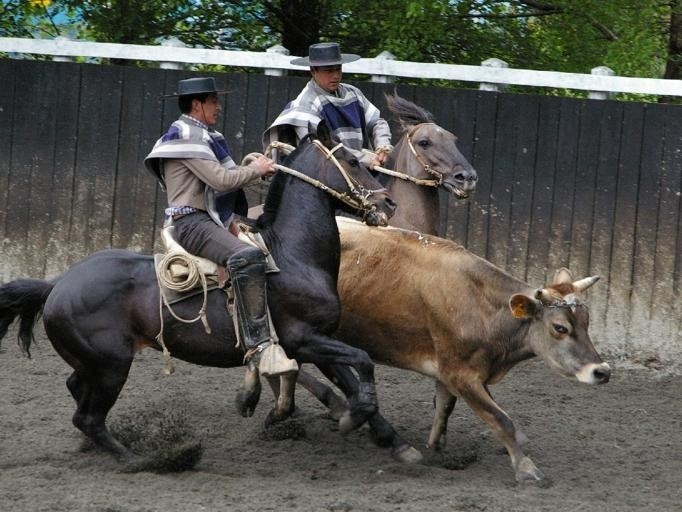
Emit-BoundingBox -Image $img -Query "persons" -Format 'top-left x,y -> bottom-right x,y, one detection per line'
154,76 -> 308,379
262,41 -> 393,190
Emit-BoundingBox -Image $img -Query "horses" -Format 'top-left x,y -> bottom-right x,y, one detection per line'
248,85 -> 479,421
0,117 -> 424,465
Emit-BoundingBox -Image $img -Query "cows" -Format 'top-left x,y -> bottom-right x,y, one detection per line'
234,200 -> 610,485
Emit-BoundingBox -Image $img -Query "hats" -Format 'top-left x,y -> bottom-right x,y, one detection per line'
288,41 -> 362,70
158,75 -> 237,102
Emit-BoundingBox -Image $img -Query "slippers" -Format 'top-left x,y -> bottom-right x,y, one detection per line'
226,245 -> 276,370
